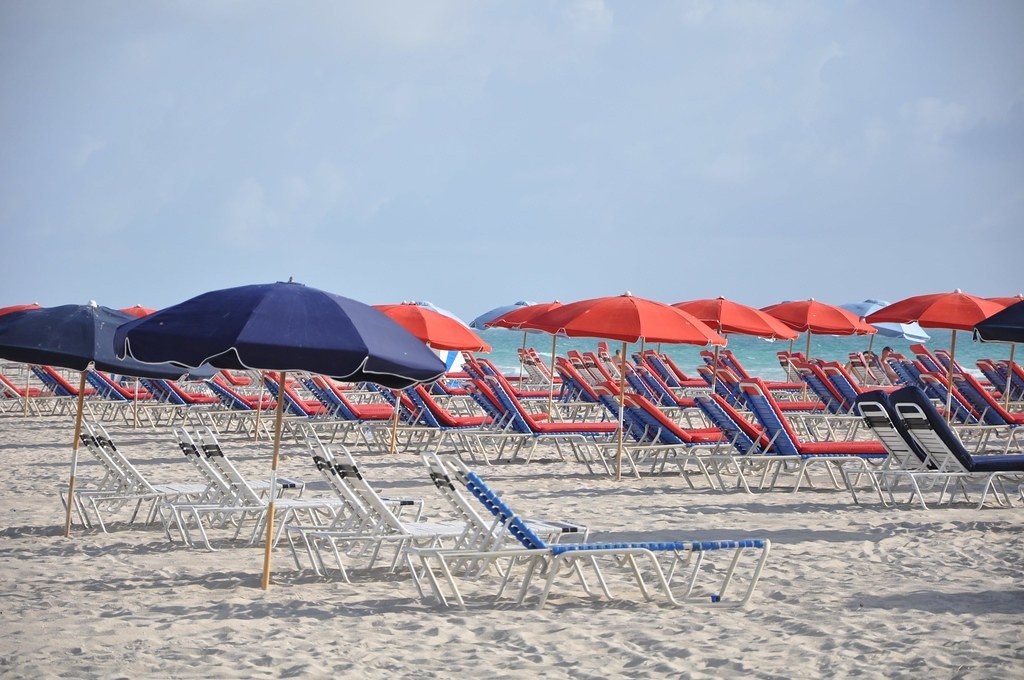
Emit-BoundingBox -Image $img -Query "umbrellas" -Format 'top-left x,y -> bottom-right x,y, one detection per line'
866,288 -> 1008,426
0,303 -> 160,538
372,302 -> 492,454
113,277 -> 446,589
759,297 -> 878,400
519,291 -> 727,482
468,299 -> 565,424
973,294 -> 1024,412
836,299 -> 931,387
670,296 -> 800,431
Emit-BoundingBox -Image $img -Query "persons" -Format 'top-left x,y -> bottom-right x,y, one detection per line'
881,347 -> 899,379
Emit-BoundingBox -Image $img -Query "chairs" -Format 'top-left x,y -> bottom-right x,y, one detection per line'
0,340 -> 1024,612
